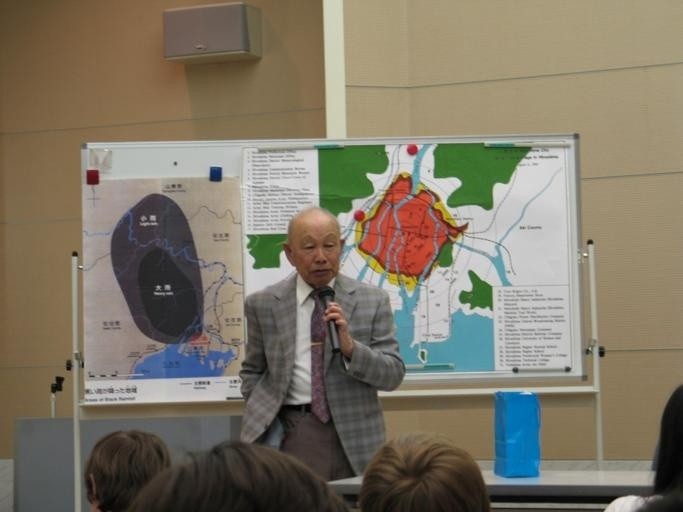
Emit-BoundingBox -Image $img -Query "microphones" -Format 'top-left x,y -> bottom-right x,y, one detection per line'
319,286 -> 341,353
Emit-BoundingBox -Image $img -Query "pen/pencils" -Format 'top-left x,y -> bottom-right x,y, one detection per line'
80,131 -> 589,410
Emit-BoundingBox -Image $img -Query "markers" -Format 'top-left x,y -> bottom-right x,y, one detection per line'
226,397 -> 244,400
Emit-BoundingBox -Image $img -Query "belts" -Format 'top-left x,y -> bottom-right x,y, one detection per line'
279,403 -> 314,414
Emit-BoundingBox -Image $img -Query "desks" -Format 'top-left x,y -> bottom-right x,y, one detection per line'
323,460 -> 680,512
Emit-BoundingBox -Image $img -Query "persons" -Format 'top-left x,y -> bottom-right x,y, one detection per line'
83,428 -> 167,512
353,432 -> 489,510
598,384 -> 683,510
127,438 -> 357,512
236,205 -> 405,482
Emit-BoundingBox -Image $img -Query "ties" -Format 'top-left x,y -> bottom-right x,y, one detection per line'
309,289 -> 333,424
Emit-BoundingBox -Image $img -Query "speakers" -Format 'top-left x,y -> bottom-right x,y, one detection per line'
161,2 -> 262,66
